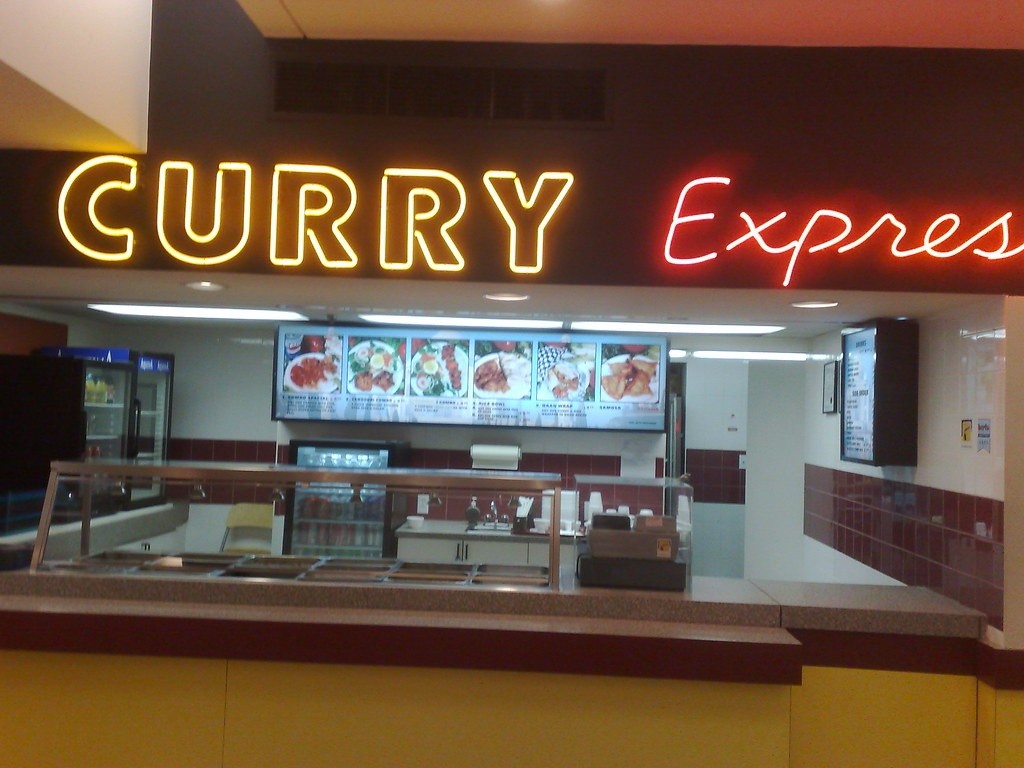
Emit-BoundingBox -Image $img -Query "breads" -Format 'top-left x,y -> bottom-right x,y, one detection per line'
601,358 -> 658,401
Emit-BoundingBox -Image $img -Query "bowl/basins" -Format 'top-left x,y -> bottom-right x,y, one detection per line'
534,520 -> 550,531
407,516 -> 424,528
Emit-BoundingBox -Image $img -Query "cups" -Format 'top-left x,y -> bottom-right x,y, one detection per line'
285,334 -> 303,358
514,517 -> 527,533
589,492 -> 653,528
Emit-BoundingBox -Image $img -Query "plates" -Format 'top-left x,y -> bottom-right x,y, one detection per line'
410,341 -> 468,397
285,352 -> 341,393
475,353 -> 531,400
601,354 -> 658,403
536,353 -> 590,401
348,340 -> 404,394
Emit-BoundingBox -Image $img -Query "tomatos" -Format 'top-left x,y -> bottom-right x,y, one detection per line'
303,335 -> 325,352
494,341 -> 517,352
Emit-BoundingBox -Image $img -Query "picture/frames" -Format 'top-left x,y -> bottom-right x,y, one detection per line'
823,360 -> 838,413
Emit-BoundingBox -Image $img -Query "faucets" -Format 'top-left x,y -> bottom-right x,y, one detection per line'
491,501 -> 498,523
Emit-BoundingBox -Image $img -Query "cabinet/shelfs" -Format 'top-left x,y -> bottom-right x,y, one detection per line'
394,517 -> 587,575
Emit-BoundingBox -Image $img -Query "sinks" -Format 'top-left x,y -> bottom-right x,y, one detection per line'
467,522 -> 509,531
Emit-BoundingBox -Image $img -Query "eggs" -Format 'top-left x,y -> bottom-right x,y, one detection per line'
423,359 -> 438,374
370,354 -> 385,369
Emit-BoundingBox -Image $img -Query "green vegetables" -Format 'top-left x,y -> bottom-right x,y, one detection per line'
348,337 -> 400,383
410,343 -> 454,397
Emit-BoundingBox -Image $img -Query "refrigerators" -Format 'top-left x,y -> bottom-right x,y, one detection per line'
39,362 -> 132,514
31,347 -> 175,510
281,436 -> 411,560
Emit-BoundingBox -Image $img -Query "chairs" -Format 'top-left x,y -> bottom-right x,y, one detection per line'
217,502 -> 273,555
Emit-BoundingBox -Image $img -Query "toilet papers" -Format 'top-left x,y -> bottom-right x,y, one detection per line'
472,444 -> 518,470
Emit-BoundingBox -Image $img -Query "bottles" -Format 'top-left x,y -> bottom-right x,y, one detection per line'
297,450 -> 387,548
85,375 -> 115,404
